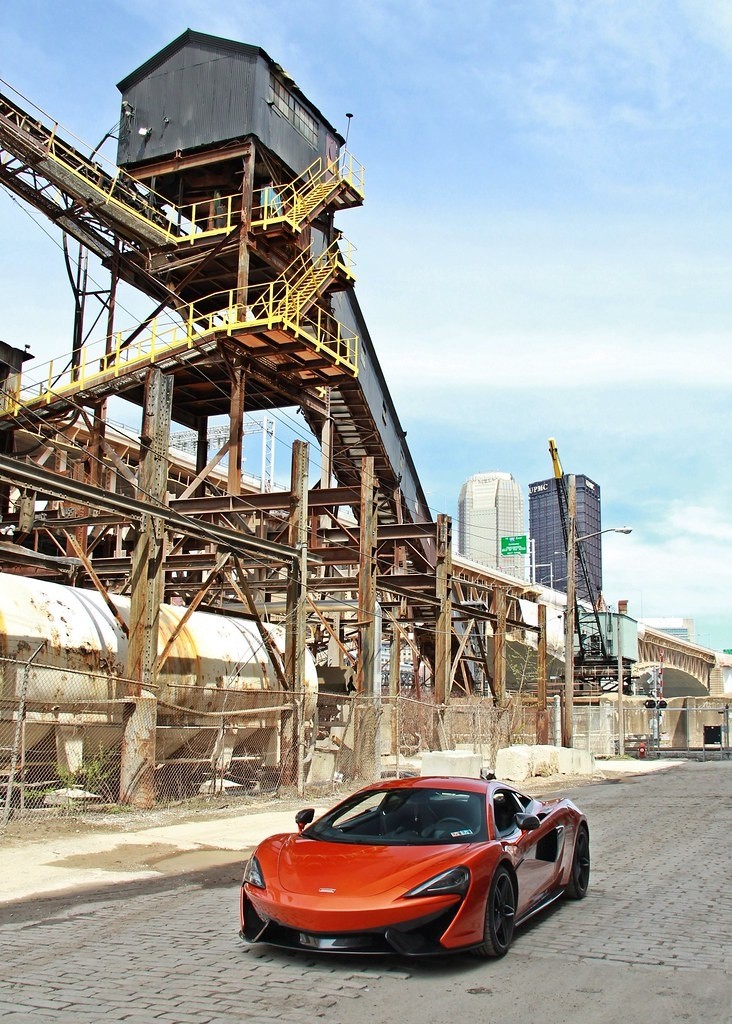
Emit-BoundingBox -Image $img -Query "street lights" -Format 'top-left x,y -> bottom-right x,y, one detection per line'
564,475 -> 632,747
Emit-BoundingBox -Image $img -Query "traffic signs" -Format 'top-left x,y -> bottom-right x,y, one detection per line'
501,536 -> 527,557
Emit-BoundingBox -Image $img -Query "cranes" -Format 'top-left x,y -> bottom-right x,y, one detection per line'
549,441 -> 633,694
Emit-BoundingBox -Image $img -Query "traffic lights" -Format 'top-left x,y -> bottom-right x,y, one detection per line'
646,700 -> 667,711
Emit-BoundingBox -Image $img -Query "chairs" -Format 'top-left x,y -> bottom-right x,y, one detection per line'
384,792 -> 437,836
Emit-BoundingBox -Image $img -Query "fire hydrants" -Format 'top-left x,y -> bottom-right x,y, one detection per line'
638,742 -> 646,759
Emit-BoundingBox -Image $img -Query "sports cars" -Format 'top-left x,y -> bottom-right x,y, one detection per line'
239,769 -> 589,969
